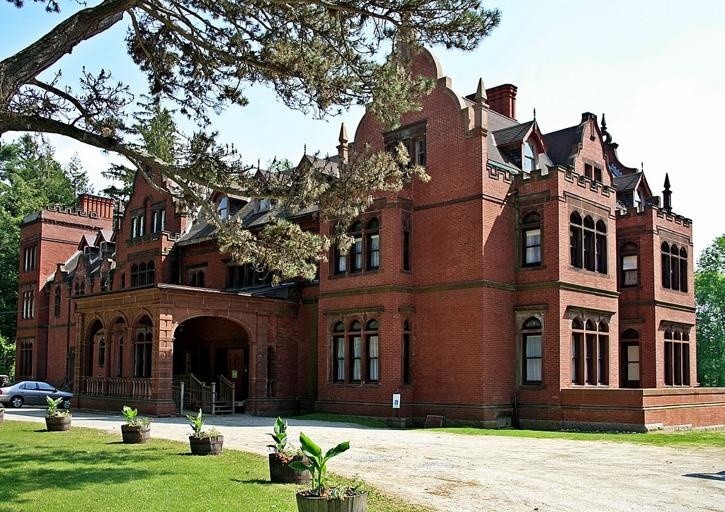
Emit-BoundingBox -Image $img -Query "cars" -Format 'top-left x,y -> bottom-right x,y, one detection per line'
0,379 -> 73,409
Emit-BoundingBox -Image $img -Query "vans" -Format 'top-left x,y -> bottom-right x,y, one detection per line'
0,374 -> 11,387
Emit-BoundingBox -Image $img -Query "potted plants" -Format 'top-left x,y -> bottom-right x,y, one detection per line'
186,408 -> 224,455
287,432 -> 367,512
44,395 -> 72,431
266,416 -> 309,485
120,405 -> 150,444
